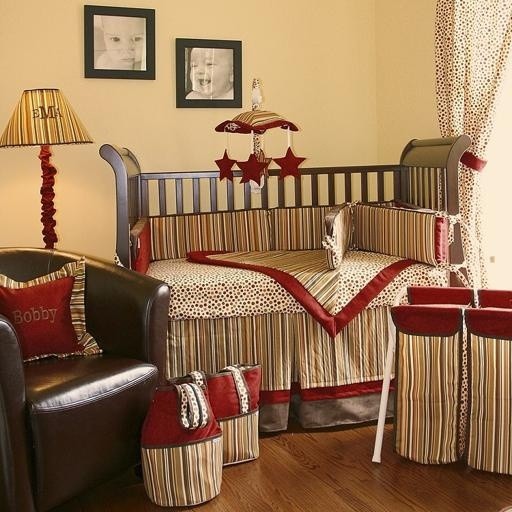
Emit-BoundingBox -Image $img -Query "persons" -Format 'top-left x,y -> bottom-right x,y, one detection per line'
185,46 -> 238,101
91,13 -> 148,72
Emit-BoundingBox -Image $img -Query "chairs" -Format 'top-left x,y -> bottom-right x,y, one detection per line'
1,247 -> 170,512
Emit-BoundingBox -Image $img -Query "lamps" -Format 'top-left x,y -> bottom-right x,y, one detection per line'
0,88 -> 93,247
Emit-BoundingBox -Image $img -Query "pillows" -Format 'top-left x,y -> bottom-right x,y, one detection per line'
1,258 -> 103,362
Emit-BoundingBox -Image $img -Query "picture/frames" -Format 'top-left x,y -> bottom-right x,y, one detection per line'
84,4 -> 157,82
175,37 -> 244,109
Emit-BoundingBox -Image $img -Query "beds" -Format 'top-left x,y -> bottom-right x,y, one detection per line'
98,134 -> 471,434
